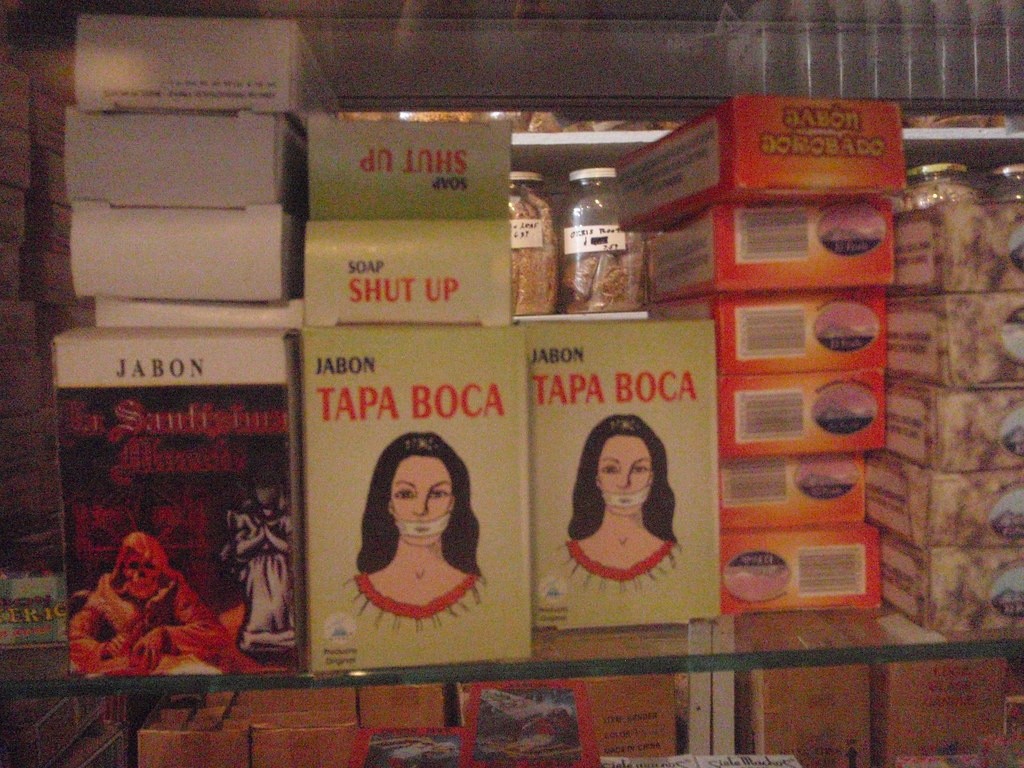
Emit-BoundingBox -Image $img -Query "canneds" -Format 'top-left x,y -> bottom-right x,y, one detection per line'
508,167 -> 646,315
890,162 -> 1024,214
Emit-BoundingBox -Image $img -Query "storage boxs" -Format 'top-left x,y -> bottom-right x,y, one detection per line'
0,12 -> 1024,767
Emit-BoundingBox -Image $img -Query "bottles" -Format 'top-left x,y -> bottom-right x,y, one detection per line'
561,167 -> 641,313
509,172 -> 557,314
